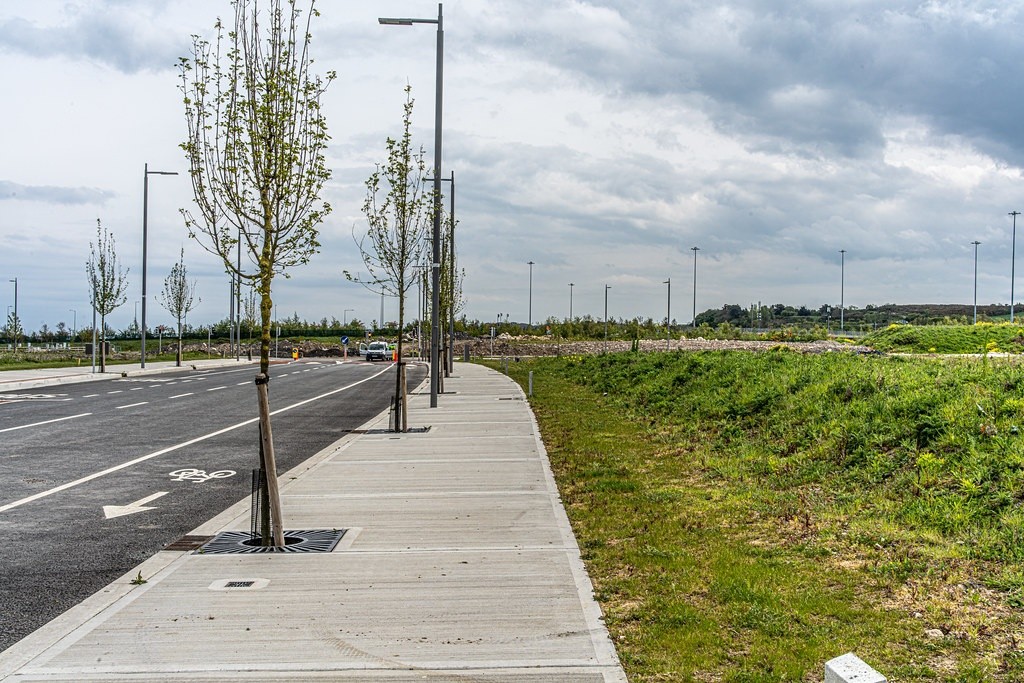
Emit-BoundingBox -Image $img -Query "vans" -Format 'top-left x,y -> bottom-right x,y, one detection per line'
359,341 -> 394,362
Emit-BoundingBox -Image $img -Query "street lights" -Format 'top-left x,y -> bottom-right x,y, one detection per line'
663,278 -> 671,350
568,282 -> 575,320
9,277 -> 18,353
140,163 -> 179,370
378,2 -> 444,409
236,228 -> 261,361
691,247 -> 700,327
421,170 -> 455,373
423,233 -> 447,370
971,241 -> 982,323
1008,211 -> 1021,323
527,261 -> 535,325
838,249 -> 847,331
604,284 -> 612,351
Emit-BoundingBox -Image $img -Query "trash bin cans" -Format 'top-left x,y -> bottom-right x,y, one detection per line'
85,343 -> 93,355
99,341 -> 110,355
292,348 -> 299,361
